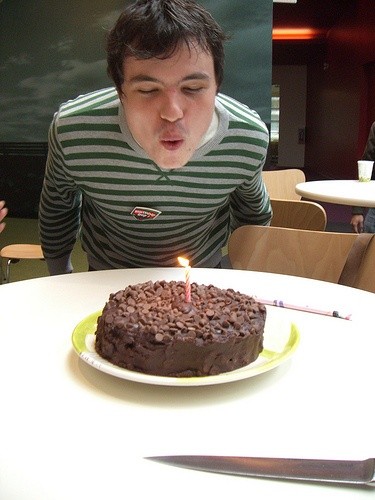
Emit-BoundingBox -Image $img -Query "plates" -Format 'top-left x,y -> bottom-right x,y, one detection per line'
73,310 -> 299,387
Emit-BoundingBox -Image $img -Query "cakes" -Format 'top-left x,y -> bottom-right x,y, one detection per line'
95,281 -> 266,378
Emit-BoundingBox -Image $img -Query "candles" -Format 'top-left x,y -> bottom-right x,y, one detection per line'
177,257 -> 191,302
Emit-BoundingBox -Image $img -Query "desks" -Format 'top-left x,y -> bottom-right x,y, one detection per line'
295,180 -> 375,207
0,268 -> 375,500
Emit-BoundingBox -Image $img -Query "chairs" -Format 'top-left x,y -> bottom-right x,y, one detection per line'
228,169 -> 375,294
1,244 -> 45,283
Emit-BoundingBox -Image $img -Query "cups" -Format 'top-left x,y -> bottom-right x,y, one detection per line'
357,161 -> 374,180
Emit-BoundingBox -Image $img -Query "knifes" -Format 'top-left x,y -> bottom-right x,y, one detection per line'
141,455 -> 375,486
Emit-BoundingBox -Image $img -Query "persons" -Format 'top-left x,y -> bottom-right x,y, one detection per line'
0,200 -> 8,234
38,0 -> 274,275
351,122 -> 375,233
